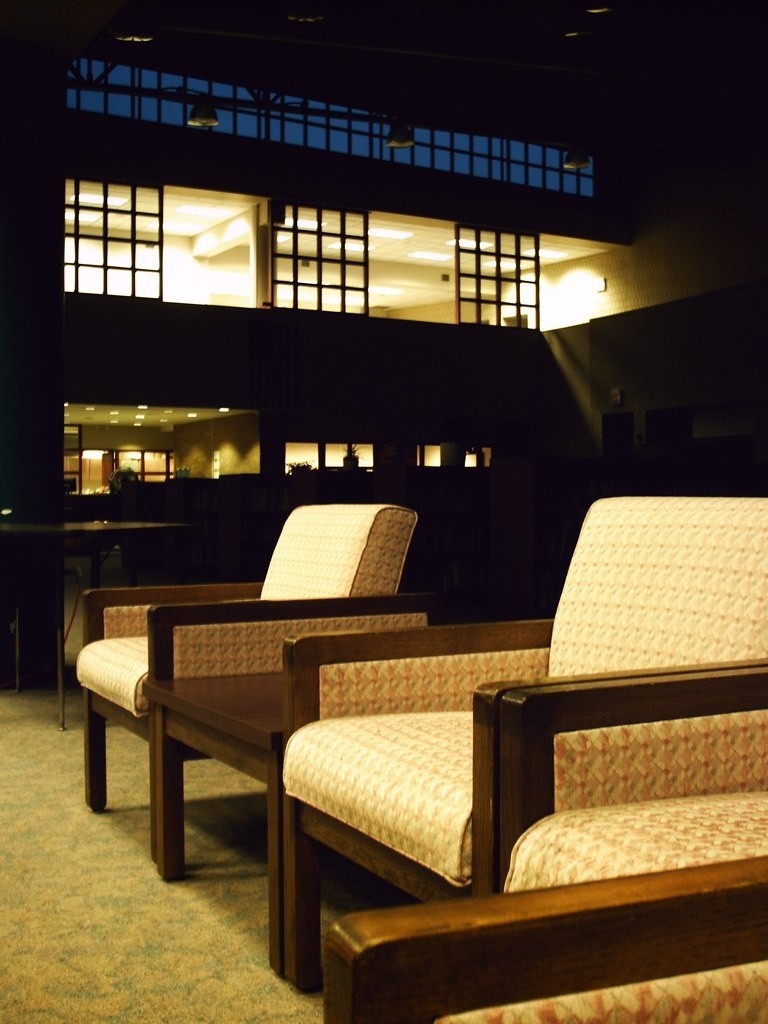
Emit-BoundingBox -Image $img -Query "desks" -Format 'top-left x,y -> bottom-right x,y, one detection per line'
0,522 -> 194,731
144,671 -> 289,978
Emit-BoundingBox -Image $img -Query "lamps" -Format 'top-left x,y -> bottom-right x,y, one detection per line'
563,150 -> 590,168
187,93 -> 218,126
386,126 -> 414,147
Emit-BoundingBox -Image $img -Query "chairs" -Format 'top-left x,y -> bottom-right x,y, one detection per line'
76,503 -> 417,862
329,855 -> 768,1024
284,497 -> 768,991
497,666 -> 767,893
